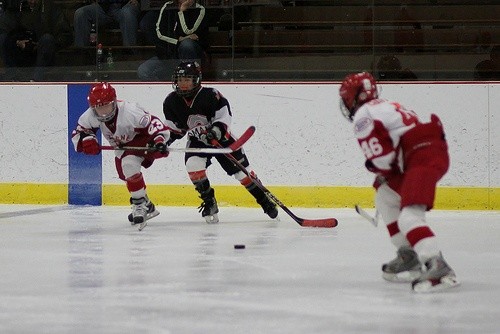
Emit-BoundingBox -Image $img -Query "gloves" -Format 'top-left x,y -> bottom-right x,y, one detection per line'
153,135 -> 169,157
81,136 -> 100,156
200,121 -> 226,147
147,130 -> 177,147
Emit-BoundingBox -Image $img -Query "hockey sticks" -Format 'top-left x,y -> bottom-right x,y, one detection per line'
99,125 -> 256,154
210,137 -> 338,228
354,204 -> 379,228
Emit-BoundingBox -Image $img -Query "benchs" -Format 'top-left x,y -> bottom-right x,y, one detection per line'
42,0 -> 500,80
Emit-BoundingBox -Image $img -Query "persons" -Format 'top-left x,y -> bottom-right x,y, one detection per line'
138,0 -> 207,81
163,61 -> 280,223
72,80 -> 170,231
339,71 -> 460,291
0,0 -> 156,66
377,56 -> 417,79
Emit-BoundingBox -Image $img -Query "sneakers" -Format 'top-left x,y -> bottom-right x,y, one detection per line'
128,194 -> 160,225
257,196 -> 280,222
197,188 -> 219,223
382,246 -> 423,283
412,252 -> 465,293
131,197 -> 147,231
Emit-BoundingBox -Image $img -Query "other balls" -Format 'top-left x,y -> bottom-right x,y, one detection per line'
235,245 -> 245,249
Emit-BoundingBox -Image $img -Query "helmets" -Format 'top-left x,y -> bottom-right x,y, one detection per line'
171,61 -> 201,98
87,81 -> 117,122
377,55 -> 401,80
340,72 -> 378,115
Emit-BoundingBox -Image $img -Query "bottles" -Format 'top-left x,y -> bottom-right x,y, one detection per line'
106,48 -> 113,72
89,24 -> 96,47
97,43 -> 104,71
